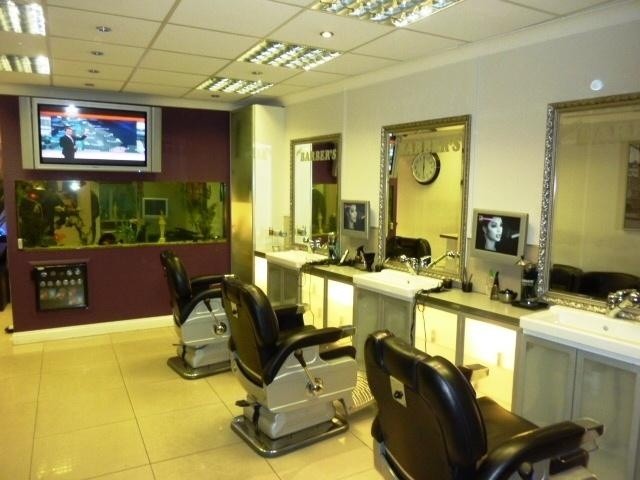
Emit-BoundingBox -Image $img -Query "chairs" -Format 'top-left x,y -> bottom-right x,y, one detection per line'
576,271 -> 640,305
160,249 -> 240,379
386,235 -> 432,267
550,264 -> 581,296
221,277 -> 358,458
364,329 -> 603,480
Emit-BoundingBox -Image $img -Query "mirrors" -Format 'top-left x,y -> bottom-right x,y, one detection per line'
377,114 -> 471,285
535,91 -> 640,322
289,133 -> 342,255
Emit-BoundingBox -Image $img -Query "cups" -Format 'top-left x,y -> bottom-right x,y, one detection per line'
443,278 -> 453,289
462,280 -> 472,292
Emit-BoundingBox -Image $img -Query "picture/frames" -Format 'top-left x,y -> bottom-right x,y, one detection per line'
622,140 -> 640,231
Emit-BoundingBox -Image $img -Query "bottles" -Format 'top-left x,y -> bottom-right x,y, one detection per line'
519,263 -> 538,303
485,270 -> 501,301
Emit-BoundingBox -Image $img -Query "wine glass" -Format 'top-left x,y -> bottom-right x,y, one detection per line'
364,252 -> 376,273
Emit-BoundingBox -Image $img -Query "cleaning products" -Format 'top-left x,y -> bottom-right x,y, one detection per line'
355,245 -> 364,263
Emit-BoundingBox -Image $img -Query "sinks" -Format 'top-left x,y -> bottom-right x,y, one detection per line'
353,268 -> 443,304
265,250 -> 328,271
519,303 -> 640,368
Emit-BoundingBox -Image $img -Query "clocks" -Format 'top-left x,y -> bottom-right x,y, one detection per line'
411,152 -> 440,185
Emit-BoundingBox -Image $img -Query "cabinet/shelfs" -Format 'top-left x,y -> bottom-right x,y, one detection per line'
511,329 -> 640,480
353,287 -> 415,373
266,262 -> 302,307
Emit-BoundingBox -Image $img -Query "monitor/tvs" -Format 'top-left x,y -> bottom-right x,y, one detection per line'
142,198 -> 168,218
18,96 -> 162,173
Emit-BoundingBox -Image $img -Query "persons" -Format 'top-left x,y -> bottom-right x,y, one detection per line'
345,204 -> 357,229
1,185 -> 9,311
59,128 -> 87,160
15,180 -> 98,246
481,215 -> 503,252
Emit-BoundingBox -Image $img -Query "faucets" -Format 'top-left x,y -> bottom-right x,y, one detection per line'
303,237 -> 314,254
607,292 -> 639,317
423,251 -> 450,268
399,254 -> 420,276
616,288 -> 636,298
320,241 -> 328,249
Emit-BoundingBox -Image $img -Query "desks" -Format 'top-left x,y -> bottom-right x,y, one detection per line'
440,232 -> 458,265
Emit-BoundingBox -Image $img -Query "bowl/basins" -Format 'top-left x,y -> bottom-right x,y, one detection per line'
497,292 -> 517,304
